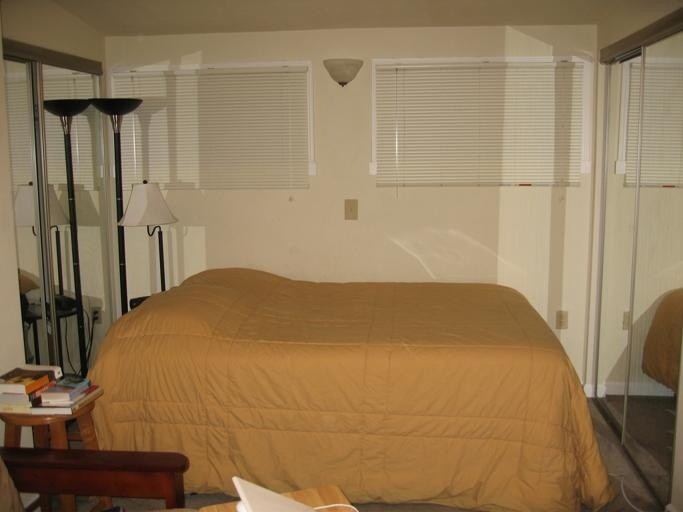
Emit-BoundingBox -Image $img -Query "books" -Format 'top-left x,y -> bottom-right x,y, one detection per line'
0,364 -> 104,416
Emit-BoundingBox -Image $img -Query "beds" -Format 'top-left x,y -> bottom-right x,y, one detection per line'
85,267 -> 615,512
640,286 -> 683,392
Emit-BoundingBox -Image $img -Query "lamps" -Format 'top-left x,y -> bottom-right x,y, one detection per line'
42,98 -> 90,383
323,58 -> 364,88
88,96 -> 143,315
13,181 -> 69,294
115,180 -> 180,293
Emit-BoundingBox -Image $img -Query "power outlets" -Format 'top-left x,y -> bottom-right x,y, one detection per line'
90,307 -> 102,325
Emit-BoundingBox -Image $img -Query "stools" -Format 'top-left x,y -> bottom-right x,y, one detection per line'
0,401 -> 113,512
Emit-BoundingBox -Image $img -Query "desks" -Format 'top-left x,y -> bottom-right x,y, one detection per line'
198,484 -> 356,512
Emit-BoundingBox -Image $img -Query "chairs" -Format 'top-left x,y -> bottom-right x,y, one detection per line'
0,446 -> 190,512
